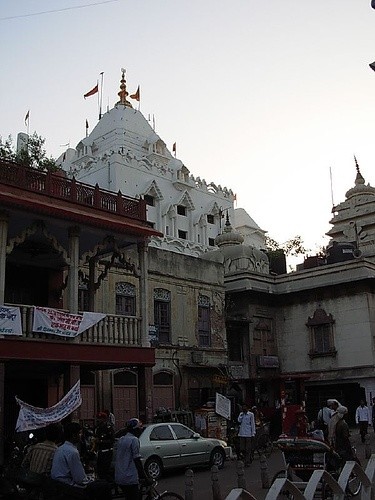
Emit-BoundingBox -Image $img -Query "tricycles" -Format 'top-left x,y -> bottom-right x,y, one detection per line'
269,438 -> 363,500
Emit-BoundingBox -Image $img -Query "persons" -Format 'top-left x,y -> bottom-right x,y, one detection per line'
115,417 -> 150,500
0,385 -> 375,500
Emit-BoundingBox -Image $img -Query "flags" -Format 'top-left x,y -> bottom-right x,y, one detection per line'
15,379 -> 83,433
84,84 -> 98,96
173,143 -> 176,151
130,87 -> 140,101
86,120 -> 89,128
32,305 -> 106,338
0,306 -> 23,336
25,111 -> 29,121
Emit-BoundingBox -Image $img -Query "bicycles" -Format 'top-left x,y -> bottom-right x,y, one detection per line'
110,476 -> 185,500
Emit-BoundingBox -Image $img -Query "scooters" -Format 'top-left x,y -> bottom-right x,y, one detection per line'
9,423 -> 98,490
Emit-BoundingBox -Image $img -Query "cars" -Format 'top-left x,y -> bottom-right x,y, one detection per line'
103,422 -> 227,481
